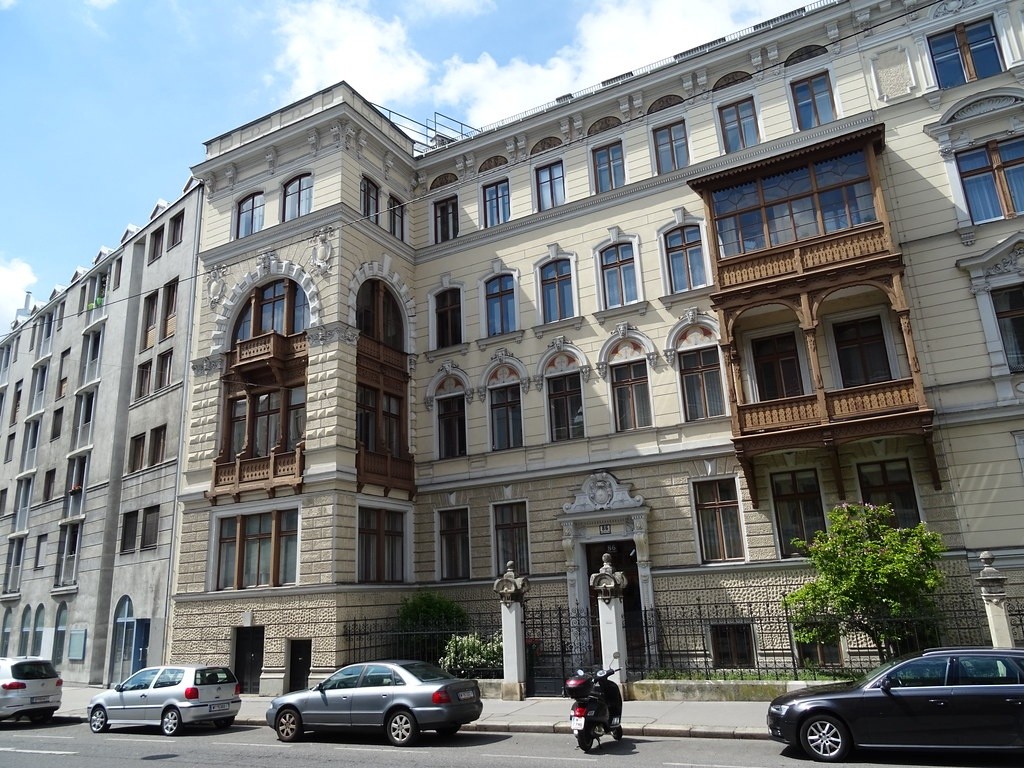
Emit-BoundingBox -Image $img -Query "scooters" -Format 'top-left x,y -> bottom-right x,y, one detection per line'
565,651 -> 623,753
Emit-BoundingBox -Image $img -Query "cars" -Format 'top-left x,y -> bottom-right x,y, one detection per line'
0,655 -> 63,730
87,663 -> 243,737
265,660 -> 483,747
766,646 -> 1024,768
